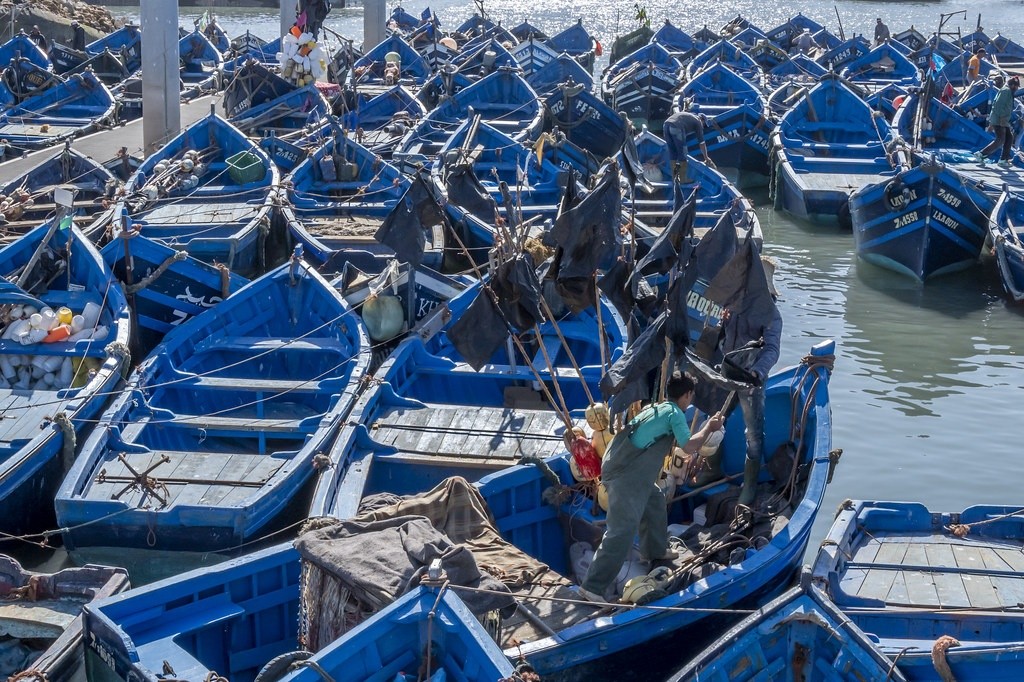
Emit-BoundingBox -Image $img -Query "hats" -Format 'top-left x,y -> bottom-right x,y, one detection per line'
71,20 -> 80,26
977,48 -> 988,54
698,113 -> 707,121
1008,77 -> 1021,87
876,18 -> 882,22
803,29 -> 811,35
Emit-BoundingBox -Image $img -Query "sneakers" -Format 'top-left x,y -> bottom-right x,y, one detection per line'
639,549 -> 679,560
998,159 -> 1012,167
576,586 -> 608,608
974,151 -> 987,165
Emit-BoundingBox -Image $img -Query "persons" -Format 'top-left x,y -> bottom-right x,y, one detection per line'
576,371 -> 724,609
966,47 -> 988,84
70,20 -> 85,51
874,17 -> 890,47
689,259 -> 783,514
29,24 -> 47,52
663,112 -> 711,183
974,75 -> 1021,166
792,27 -> 822,57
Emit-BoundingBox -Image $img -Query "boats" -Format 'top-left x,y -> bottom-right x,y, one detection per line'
599,8 -> 1024,316
810,500 -> 1024,681
0,544 -> 244,682
257,559 -> 544,682
0,2 -> 782,551
664,563 -> 910,682
82,338 -> 854,682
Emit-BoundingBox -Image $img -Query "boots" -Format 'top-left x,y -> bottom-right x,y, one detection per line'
671,160 -> 676,179
680,161 -> 694,183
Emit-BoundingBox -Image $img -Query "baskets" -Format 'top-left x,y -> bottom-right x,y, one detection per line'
225,150 -> 265,184
